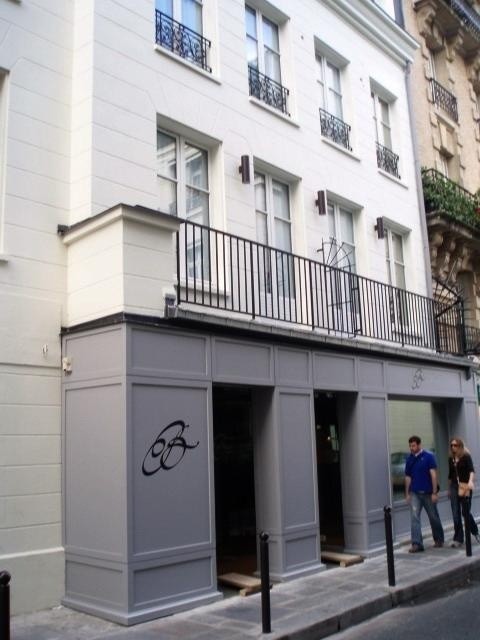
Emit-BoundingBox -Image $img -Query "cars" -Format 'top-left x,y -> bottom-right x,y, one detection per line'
390,451 -> 411,488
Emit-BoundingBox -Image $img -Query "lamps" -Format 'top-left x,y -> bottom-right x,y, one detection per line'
315,191 -> 326,215
375,217 -> 384,239
238,154 -> 250,185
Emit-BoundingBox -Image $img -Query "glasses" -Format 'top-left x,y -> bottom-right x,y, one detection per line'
451,444 -> 457,447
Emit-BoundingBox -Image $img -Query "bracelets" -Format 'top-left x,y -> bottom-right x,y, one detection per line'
432,492 -> 437,494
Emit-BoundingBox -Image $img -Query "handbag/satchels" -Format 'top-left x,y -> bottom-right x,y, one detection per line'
458,482 -> 470,497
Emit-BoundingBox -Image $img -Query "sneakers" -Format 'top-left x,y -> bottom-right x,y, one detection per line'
452,541 -> 461,548
475,533 -> 480,542
434,540 -> 442,547
409,544 -> 423,553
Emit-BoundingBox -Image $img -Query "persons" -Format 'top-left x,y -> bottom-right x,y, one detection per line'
404,435 -> 444,554
447,437 -> 480,549
